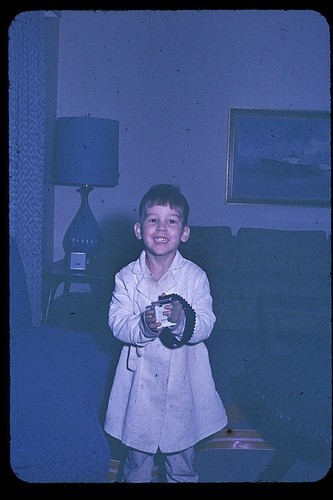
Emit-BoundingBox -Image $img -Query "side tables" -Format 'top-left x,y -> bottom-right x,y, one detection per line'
34,260 -> 118,353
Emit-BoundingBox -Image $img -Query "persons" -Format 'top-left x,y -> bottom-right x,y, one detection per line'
102,183 -> 228,483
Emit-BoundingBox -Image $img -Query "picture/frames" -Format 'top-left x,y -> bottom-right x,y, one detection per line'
68,251 -> 86,272
222,105 -> 331,214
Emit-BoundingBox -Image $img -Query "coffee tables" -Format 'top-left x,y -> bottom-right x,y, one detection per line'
220,374 -> 320,481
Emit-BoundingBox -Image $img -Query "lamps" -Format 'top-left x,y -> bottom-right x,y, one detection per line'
49,113 -> 120,265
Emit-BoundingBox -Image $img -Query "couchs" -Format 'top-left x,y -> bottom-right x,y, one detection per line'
102,222 -> 331,391
